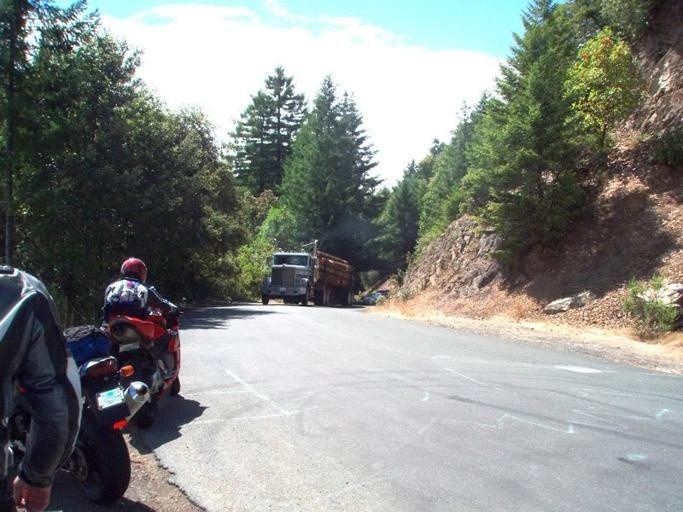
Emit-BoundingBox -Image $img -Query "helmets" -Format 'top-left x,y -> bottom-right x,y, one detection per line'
120,257 -> 147,281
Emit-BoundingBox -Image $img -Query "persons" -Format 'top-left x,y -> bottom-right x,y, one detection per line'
0,260 -> 84,511
101,256 -> 182,320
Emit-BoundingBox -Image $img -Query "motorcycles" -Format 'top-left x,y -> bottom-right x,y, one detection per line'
7,298 -> 184,505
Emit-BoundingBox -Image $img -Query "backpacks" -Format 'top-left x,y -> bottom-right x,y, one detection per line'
104,279 -> 148,314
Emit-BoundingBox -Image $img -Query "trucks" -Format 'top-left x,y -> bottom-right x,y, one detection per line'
259,251 -> 356,307
